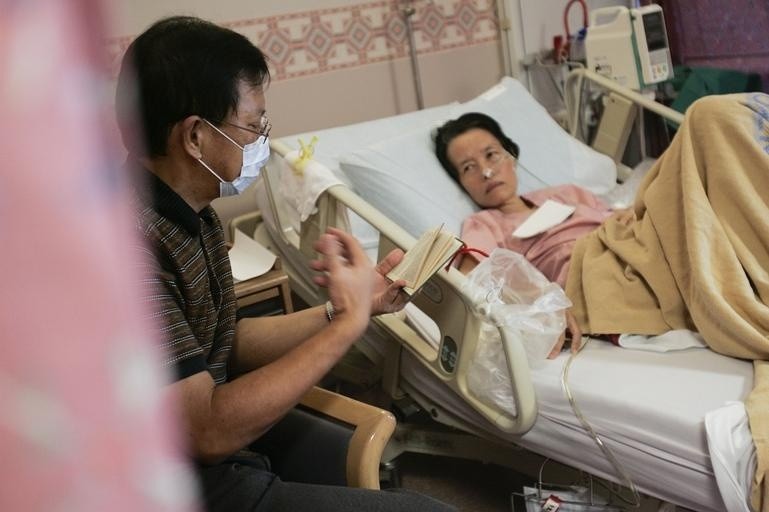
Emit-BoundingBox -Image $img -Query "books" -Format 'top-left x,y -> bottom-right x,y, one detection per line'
383,222 -> 465,302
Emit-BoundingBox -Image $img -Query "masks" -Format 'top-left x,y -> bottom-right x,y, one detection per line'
195,119 -> 271,198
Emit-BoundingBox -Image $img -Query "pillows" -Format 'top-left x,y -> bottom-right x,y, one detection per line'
336,74 -> 618,242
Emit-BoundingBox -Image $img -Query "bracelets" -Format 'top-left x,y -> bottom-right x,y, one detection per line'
325,301 -> 335,321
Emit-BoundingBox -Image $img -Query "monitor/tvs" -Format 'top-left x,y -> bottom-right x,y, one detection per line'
585,5 -> 674,90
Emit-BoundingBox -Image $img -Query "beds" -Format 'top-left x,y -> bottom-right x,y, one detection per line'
224,64 -> 769,512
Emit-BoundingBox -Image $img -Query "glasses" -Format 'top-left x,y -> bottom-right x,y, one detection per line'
222,113 -> 271,144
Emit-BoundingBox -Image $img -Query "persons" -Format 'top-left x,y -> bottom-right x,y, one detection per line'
113,15 -> 461,512
430,91 -> 769,360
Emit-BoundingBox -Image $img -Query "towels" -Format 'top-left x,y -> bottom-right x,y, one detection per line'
275,150 -> 354,237
